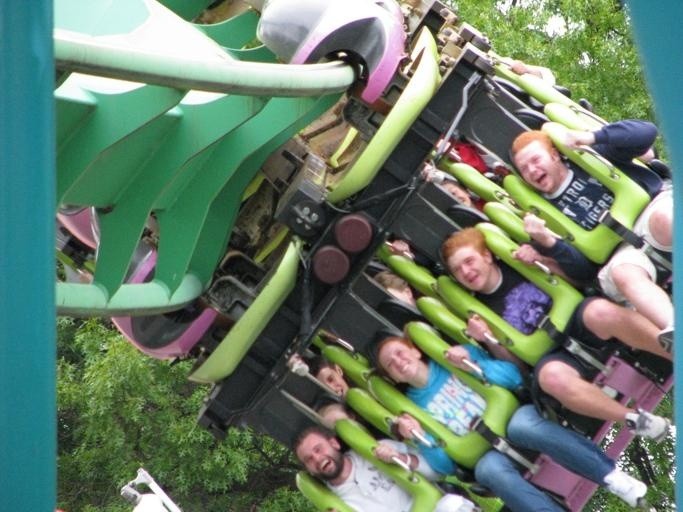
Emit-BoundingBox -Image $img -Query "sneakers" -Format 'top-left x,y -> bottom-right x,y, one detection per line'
624,408 -> 670,442
657,325 -> 674,354
603,467 -> 648,508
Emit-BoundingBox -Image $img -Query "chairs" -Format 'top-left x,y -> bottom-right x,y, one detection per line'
504,121 -> 674,285
543,101 -> 601,131
293,419 -> 444,512
493,60 -> 589,111
368,321 -> 602,512
435,223 -> 664,463
312,332 -> 373,387
440,160 -> 527,218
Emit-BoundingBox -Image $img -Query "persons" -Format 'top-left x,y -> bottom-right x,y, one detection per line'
373,229 -> 673,443
296,357 -> 476,512
420,61 -> 673,355
373,313 -> 647,512
